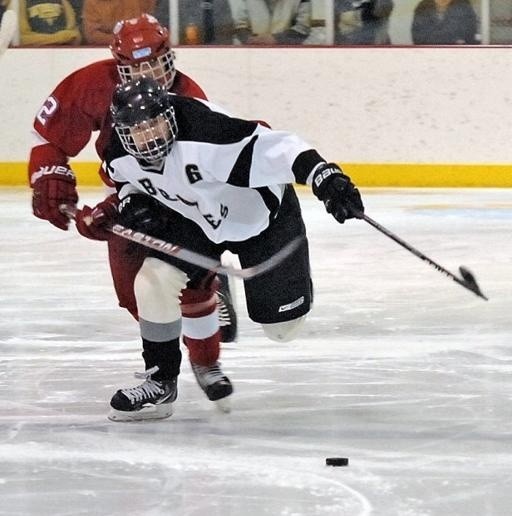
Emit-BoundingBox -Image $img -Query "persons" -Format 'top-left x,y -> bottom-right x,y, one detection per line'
91,76 -> 365,412
157,0 -> 235,46
335,0 -> 396,46
19,0 -> 82,46
228,1 -> 313,44
25,13 -> 274,402
411,0 -> 481,46
1,0 -> 18,58
83,0 -> 159,46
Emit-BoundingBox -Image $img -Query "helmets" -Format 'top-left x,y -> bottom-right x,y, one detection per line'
109,74 -> 178,163
108,13 -> 176,90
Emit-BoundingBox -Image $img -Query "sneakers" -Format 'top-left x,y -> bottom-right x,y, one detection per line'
111,365 -> 177,411
215,274 -> 236,342
191,361 -> 232,400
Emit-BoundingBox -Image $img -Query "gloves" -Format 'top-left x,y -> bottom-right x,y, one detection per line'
312,164 -> 364,223
31,165 -> 77,230
76,193 -> 121,241
118,193 -> 159,229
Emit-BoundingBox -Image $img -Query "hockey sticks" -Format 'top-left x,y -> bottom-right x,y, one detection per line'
59,203 -> 302,279
342,197 -> 490,302
